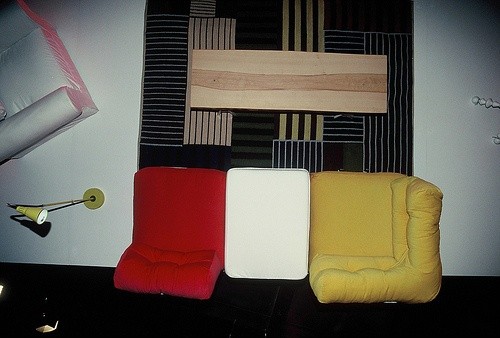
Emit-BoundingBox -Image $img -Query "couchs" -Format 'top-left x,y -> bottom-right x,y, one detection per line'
0,0 -> 99,162
113,166 -> 227,300
310,171 -> 443,304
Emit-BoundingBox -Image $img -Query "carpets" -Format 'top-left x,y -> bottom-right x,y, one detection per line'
136,0 -> 414,177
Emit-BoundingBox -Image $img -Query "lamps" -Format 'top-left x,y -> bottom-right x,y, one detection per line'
6,188 -> 105,225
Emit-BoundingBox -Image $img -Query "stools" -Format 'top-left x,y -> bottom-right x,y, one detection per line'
224,168 -> 310,280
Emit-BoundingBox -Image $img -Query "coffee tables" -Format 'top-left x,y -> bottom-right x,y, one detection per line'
189,49 -> 388,116
